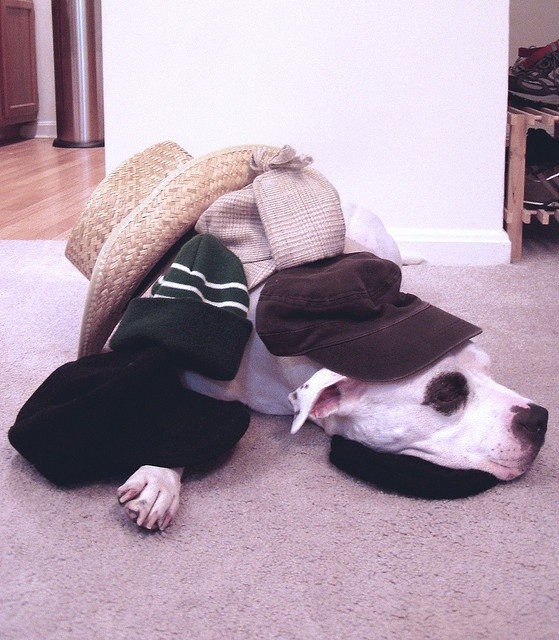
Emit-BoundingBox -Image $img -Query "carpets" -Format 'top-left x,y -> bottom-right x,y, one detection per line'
0,238 -> 558,640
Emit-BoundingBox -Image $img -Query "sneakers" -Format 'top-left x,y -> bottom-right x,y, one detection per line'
506,164 -> 559,211
508,41 -> 559,106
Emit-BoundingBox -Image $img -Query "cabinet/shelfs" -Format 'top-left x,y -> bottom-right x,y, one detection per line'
0,0 -> 36,127
504,100 -> 559,263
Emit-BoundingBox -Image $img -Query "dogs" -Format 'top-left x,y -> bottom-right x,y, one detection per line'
116,227 -> 551,532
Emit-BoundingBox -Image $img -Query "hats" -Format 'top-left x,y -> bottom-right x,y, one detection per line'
6,352 -> 251,488
104,234 -> 252,380
193,164 -> 346,291
64,141 -> 281,361
254,251 -> 482,381
